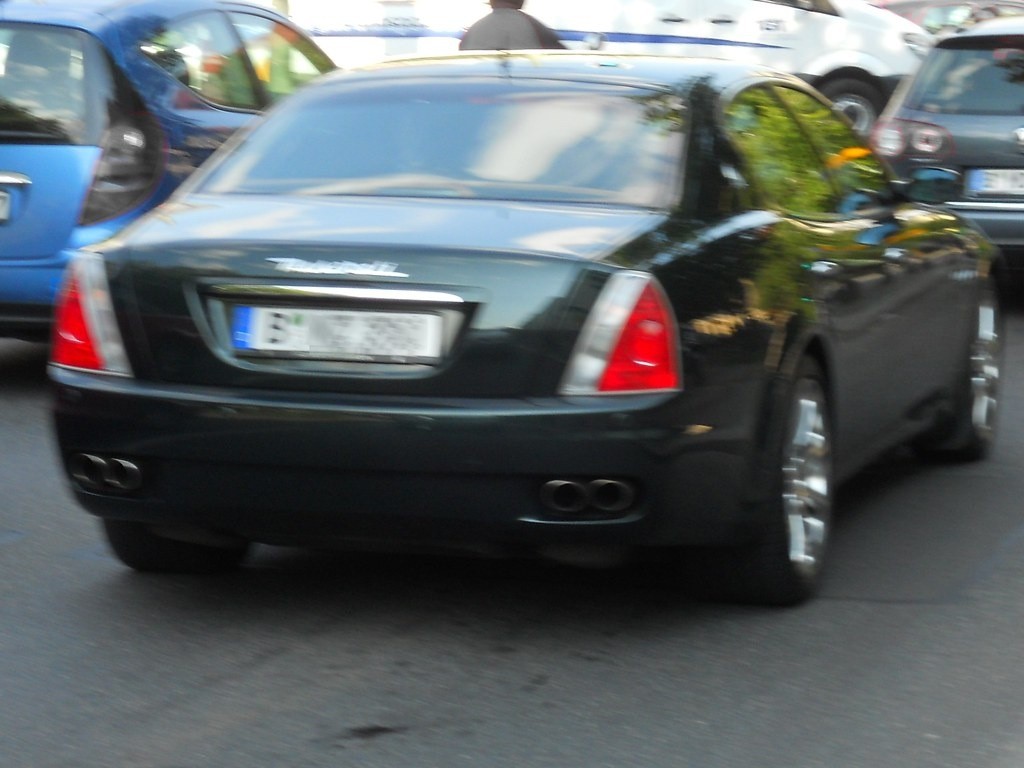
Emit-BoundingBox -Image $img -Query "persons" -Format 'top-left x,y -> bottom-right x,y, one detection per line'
459,0 -> 569,51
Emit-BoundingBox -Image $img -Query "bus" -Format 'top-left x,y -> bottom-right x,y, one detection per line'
196,1 -> 940,141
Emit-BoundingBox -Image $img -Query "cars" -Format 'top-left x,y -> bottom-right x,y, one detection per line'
858,16 -> 1022,256
0,2 -> 337,340
47,48 -> 1003,611
